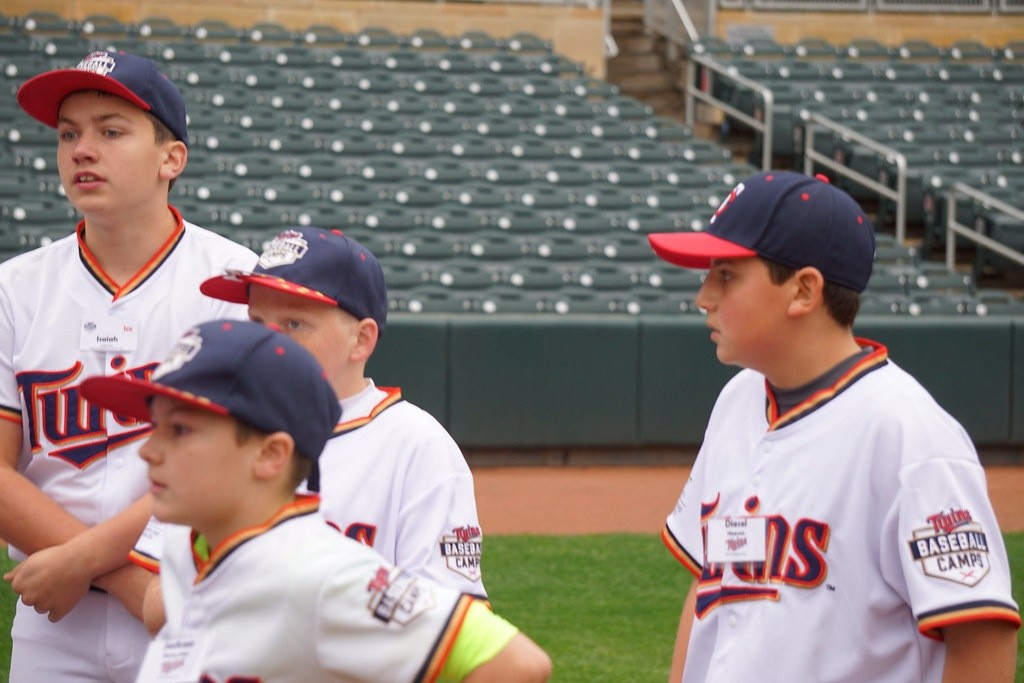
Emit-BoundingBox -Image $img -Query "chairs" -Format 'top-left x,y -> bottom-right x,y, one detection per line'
0,14 -> 1024,318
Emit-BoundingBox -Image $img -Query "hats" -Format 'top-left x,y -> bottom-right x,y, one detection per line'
200,225 -> 387,334
16,49 -> 190,147
80,319 -> 343,459
650,171 -> 876,293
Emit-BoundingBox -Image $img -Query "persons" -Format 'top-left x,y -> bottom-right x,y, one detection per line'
80,320 -> 552,683
648,171 -> 1022,682
0,50 -> 260,683
128,226 -> 491,641
